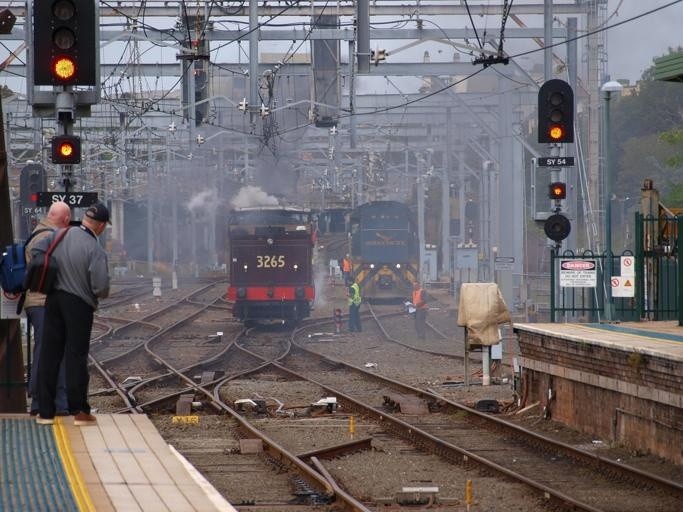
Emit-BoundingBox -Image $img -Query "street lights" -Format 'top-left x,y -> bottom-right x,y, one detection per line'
598,73 -> 626,322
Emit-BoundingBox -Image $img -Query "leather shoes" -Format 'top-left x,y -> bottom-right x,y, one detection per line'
35,414 -> 54,425
74,413 -> 98,424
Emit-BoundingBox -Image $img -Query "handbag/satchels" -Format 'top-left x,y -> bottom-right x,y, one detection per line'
25,226 -> 72,295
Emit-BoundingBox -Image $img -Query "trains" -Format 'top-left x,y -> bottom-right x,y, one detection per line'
224,206 -> 345,328
344,200 -> 418,303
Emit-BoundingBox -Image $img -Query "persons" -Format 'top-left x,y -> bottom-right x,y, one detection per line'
342,254 -> 350,285
334,309 -> 343,333
346,276 -> 361,332
22,202 -> 71,414
29,202 -> 111,427
402,281 -> 427,340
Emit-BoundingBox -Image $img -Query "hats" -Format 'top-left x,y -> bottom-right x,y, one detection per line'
86,203 -> 113,227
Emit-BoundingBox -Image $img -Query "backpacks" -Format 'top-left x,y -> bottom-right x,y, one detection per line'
0,228 -> 56,293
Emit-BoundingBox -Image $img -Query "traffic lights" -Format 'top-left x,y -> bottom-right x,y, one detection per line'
19,164 -> 48,209
544,215 -> 570,241
548,179 -> 566,202
538,79 -> 574,143
31,0 -> 98,86
51,135 -> 81,164
